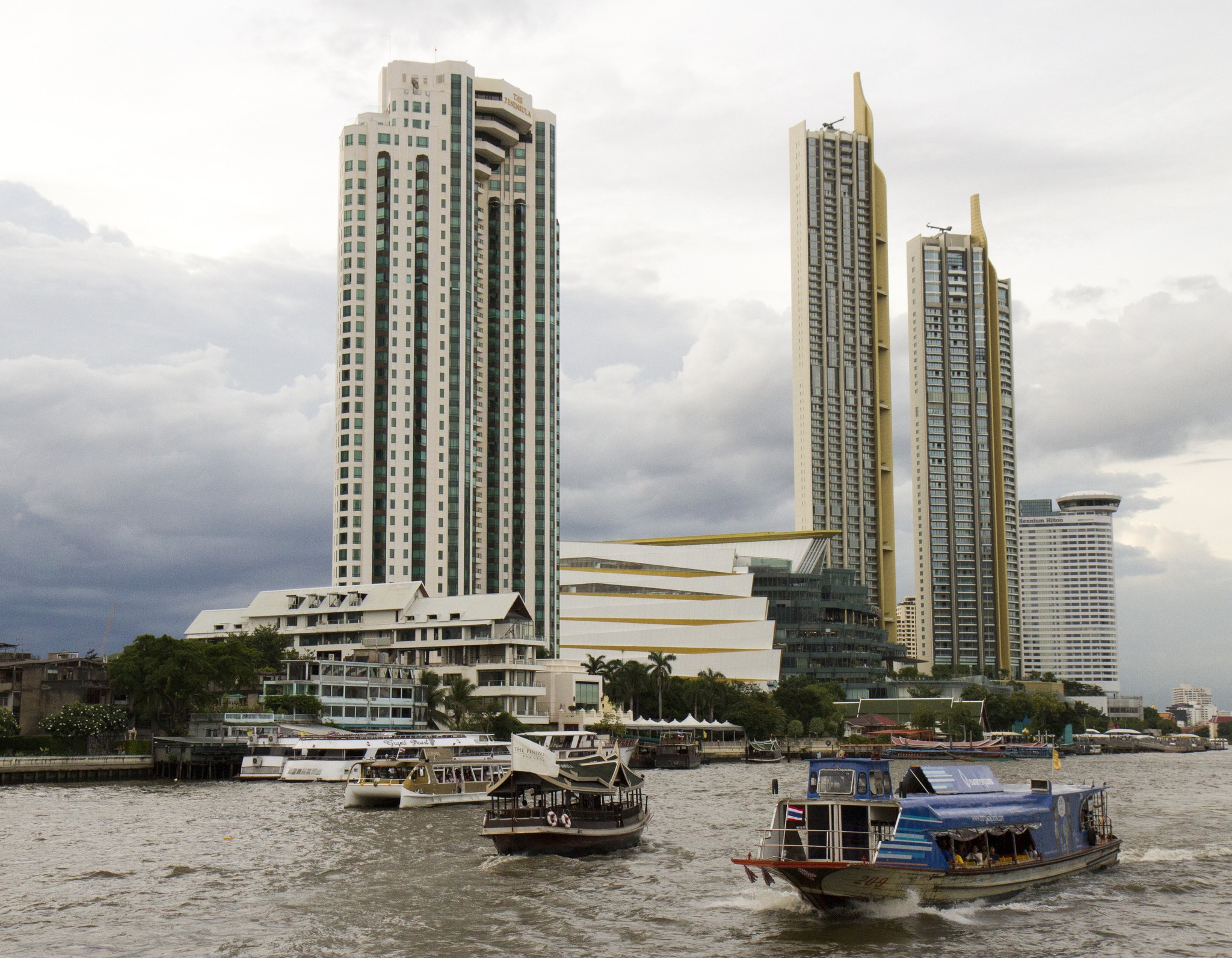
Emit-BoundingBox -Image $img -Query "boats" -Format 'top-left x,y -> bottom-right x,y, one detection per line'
477,731 -> 657,859
741,736 -> 786,763
724,745 -> 1123,918
654,728 -> 704,768
243,727 -> 640,809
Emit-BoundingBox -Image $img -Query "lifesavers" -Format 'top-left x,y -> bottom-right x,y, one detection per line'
547,811 -> 557,826
561,814 -> 571,828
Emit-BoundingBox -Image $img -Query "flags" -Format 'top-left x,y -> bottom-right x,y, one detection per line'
399,747 -> 405,753
1053,749 -> 1061,770
328,731 -> 336,736
512,734 -> 560,778
787,805 -> 803,821
491,750 -> 493,758
301,732 -> 307,736
278,731 -> 284,736
703,731 -> 707,738
247,731 -> 253,736
678,731 -> 684,738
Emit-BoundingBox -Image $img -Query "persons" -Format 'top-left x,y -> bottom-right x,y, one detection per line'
536,800 -> 545,808
564,789 -> 579,805
936,836 -> 1038,866
365,767 -> 499,794
1082,794 -> 1106,830
519,800 -> 530,808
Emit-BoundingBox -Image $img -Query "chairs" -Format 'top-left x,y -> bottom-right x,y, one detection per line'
964,855 -> 1030,865
